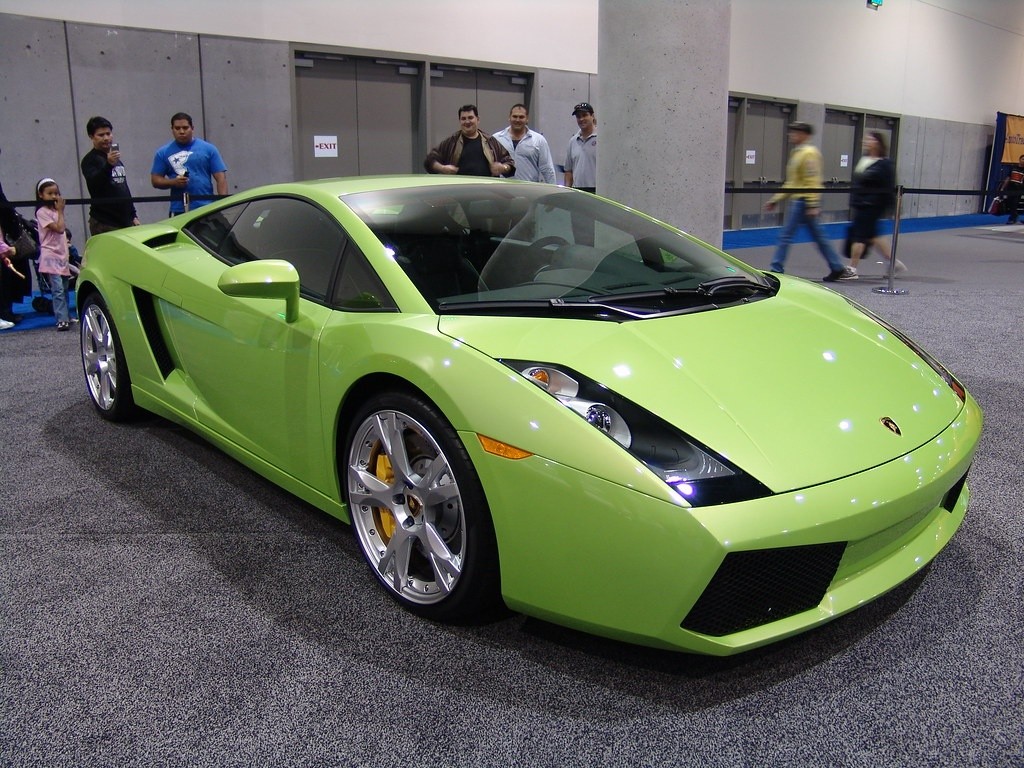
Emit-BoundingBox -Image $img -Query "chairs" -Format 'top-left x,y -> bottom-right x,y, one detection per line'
395,198 -> 511,299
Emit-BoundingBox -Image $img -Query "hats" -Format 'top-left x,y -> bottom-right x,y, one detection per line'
572,103 -> 594,116
788,120 -> 812,134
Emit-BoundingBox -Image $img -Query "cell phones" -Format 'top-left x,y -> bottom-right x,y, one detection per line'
110,143 -> 119,152
183,172 -> 189,178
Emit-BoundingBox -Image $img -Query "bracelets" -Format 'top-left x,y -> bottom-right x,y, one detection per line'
504,165 -> 508,171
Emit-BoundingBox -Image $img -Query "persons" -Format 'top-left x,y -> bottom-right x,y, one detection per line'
424,105 -> 516,229
65,229 -> 79,264
0,184 -> 36,330
35,178 -> 70,330
492,104 -> 557,235
81,116 -> 140,234
1000,155 -> 1024,224
765,121 -> 848,283
563,103 -> 597,247
151,113 -> 228,217
839,130 -> 907,280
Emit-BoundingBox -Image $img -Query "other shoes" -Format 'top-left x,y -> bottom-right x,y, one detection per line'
884,260 -> 908,279
0,317 -> 15,330
841,268 -> 857,280
1006,220 -> 1017,224
823,267 -> 847,281
57,320 -> 69,331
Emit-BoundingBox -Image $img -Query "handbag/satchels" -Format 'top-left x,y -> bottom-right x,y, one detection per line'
988,194 -> 1009,216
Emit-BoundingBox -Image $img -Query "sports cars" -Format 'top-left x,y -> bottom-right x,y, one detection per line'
75,172 -> 987,658
